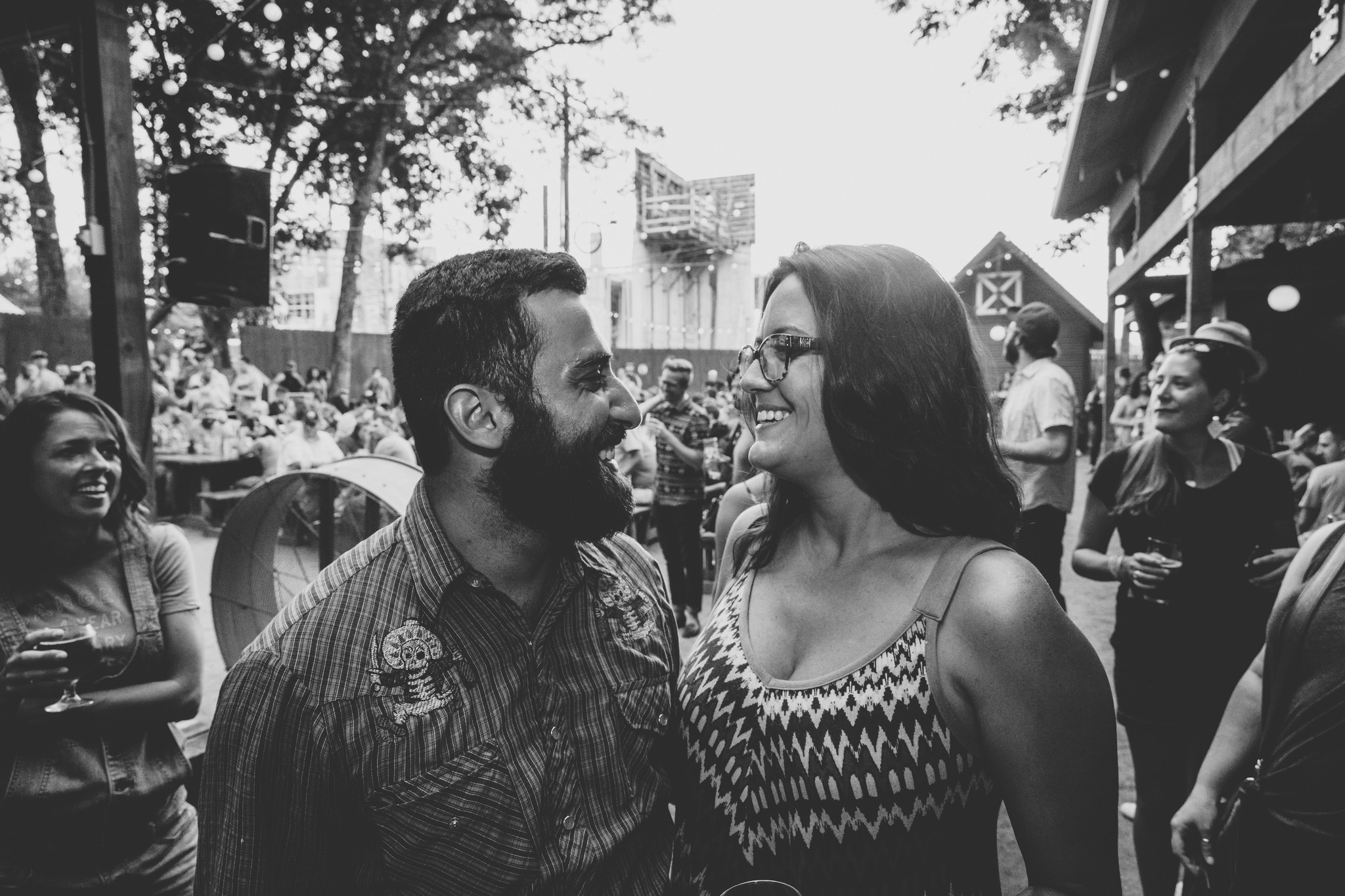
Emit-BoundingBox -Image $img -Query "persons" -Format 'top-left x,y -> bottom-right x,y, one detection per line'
674,242 -> 1127,896
1070,344 -> 1303,896
190,250 -> 684,896
1170,513 -> 1345,896
986,299 -> 1079,620
1079,320 -> 1345,536
0,333 -> 756,642
1,389 -> 209,895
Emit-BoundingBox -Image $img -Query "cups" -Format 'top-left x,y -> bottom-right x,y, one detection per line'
1143,533 -> 1172,607
720,878 -> 804,896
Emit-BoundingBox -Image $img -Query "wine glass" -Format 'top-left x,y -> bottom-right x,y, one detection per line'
33,617 -> 97,716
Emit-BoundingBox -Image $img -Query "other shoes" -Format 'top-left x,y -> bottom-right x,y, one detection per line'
674,608 -> 701,637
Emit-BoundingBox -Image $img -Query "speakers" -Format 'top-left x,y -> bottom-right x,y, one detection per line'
165,164 -> 271,309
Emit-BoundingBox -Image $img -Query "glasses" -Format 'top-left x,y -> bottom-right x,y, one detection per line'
737,332 -> 822,384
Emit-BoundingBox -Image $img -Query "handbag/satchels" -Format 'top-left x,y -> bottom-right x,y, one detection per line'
1202,770 -> 1264,896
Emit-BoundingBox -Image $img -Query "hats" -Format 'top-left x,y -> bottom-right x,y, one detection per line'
662,358 -> 694,385
1169,320 -> 1268,385
1006,300 -> 1060,344
